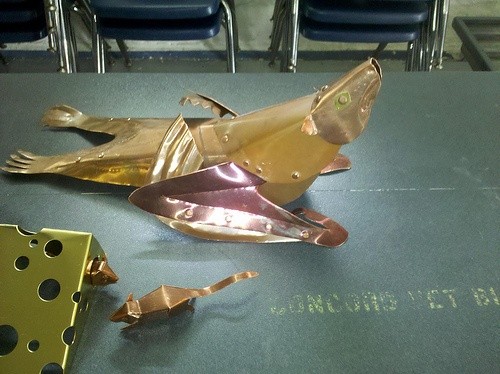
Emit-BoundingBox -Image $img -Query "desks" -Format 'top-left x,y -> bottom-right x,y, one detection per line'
0,68 -> 500,374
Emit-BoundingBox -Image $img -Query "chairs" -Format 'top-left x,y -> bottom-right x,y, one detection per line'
0,0 -> 245,74
261,0 -> 447,74
